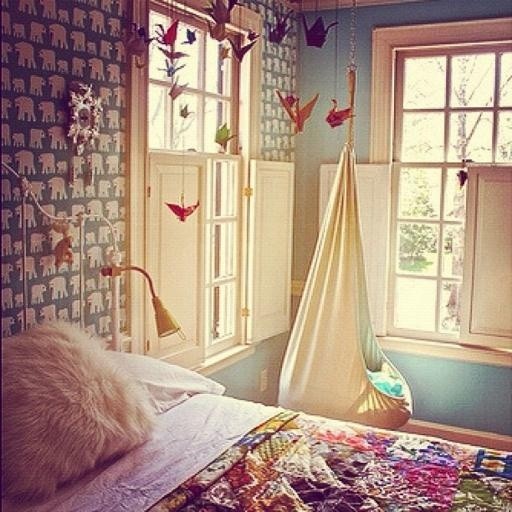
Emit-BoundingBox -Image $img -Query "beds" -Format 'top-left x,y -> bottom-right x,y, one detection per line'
0,159 -> 512,512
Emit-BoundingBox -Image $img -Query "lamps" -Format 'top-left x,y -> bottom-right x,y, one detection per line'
112,264 -> 181,338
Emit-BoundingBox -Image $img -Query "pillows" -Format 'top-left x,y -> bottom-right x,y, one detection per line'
106,350 -> 226,416
